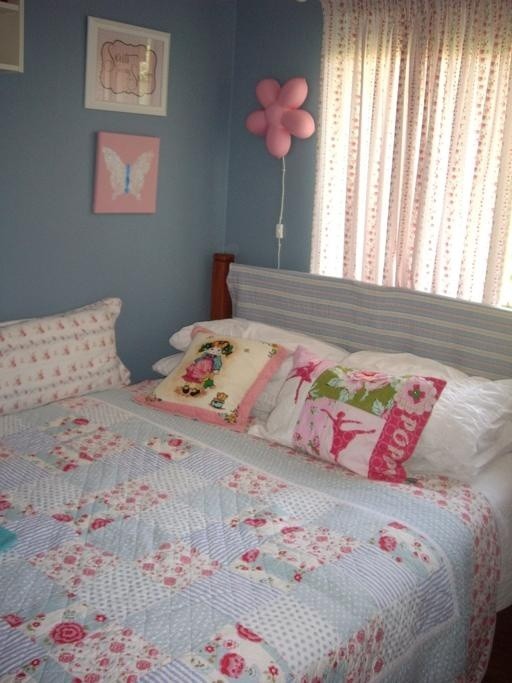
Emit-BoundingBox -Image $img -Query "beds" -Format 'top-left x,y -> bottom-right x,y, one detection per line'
0,254 -> 512,681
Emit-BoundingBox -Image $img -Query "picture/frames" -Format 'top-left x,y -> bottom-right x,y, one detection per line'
83,15 -> 170,116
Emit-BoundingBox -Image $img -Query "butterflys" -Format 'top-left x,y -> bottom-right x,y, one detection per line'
102,146 -> 154,201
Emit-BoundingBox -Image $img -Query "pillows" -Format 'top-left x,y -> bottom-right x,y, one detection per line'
1,295 -> 131,417
147,351 -> 281,420
167,318 -> 352,365
148,324 -> 289,434
246,345 -> 446,482
337,350 -> 512,482
